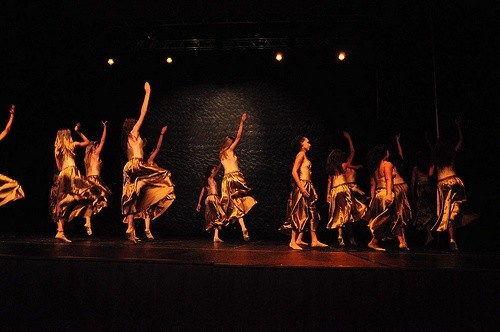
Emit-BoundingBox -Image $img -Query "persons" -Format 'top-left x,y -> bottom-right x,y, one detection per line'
48,123 -> 93,243
0,105 -> 24,206
296,230 -> 308,245
121,83 -> 174,244
287,134 -> 328,250
70,121 -> 110,237
327,118 -> 477,250
195,161 -> 224,241
146,126 -> 175,239
219,111 -> 258,240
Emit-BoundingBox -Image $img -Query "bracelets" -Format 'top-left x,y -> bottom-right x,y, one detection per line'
160,131 -> 166,136
